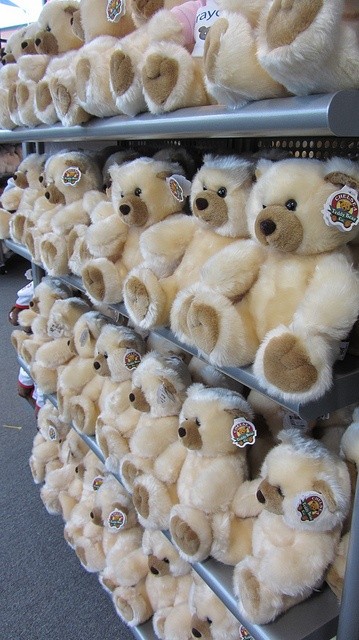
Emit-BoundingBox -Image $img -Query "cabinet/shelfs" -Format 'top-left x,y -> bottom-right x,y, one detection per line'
0,90 -> 359,640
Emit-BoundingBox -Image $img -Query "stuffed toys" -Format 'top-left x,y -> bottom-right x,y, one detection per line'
0,1 -> 359,128
0,146 -> 359,402
10,277 -> 358,626
